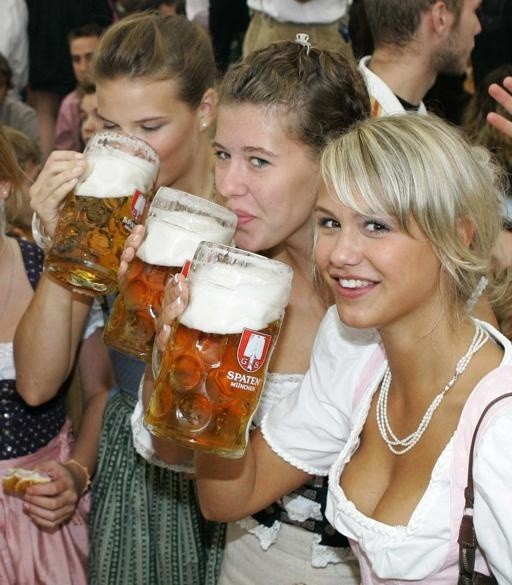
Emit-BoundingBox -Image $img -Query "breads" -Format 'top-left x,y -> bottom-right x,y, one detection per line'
2,468 -> 51,497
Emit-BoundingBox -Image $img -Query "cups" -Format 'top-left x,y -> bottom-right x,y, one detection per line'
101,183 -> 236,357
29,131 -> 163,296
142,239 -> 296,461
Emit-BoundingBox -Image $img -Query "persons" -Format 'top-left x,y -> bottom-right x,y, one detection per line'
0,0 -> 512,585
